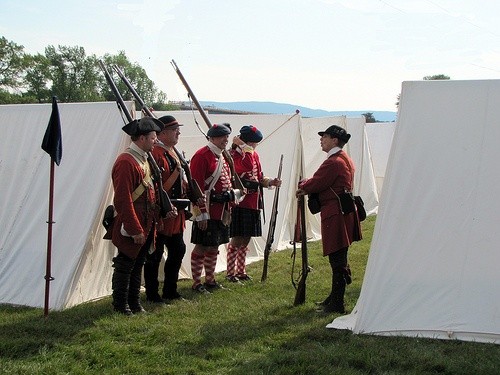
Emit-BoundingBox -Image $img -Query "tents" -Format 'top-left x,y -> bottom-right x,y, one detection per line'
301,115 -> 395,217
210,113 -> 323,251
326,79 -> 500,344
0,101 -> 136,310
135,110 -> 264,280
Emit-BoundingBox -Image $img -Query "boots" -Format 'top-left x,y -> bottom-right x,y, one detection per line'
315,279 -> 346,313
316,292 -> 333,305
131,272 -> 146,314
112,271 -> 133,316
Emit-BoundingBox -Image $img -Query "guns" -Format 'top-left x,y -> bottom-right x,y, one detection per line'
293,176 -> 311,306
112,64 -> 204,206
97,59 -> 174,220
261,154 -> 284,282
170,59 -> 245,196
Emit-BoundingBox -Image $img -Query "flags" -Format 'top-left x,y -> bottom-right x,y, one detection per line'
41,99 -> 62,166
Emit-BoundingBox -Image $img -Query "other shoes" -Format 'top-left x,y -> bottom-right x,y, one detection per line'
192,283 -> 213,295
239,275 -> 253,281
204,279 -> 230,291
178,296 -> 190,303
156,298 -> 170,305
226,276 -> 244,286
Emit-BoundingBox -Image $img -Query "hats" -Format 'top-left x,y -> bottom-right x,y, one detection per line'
207,123 -> 231,137
318,125 -> 351,143
240,125 -> 263,143
121,117 -> 166,136
158,115 -> 184,128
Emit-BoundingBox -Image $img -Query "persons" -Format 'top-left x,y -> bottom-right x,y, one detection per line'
225,125 -> 282,282
112,118 -> 176,315
296,124 -> 363,313
190,123 -> 235,294
144,116 -> 206,305
